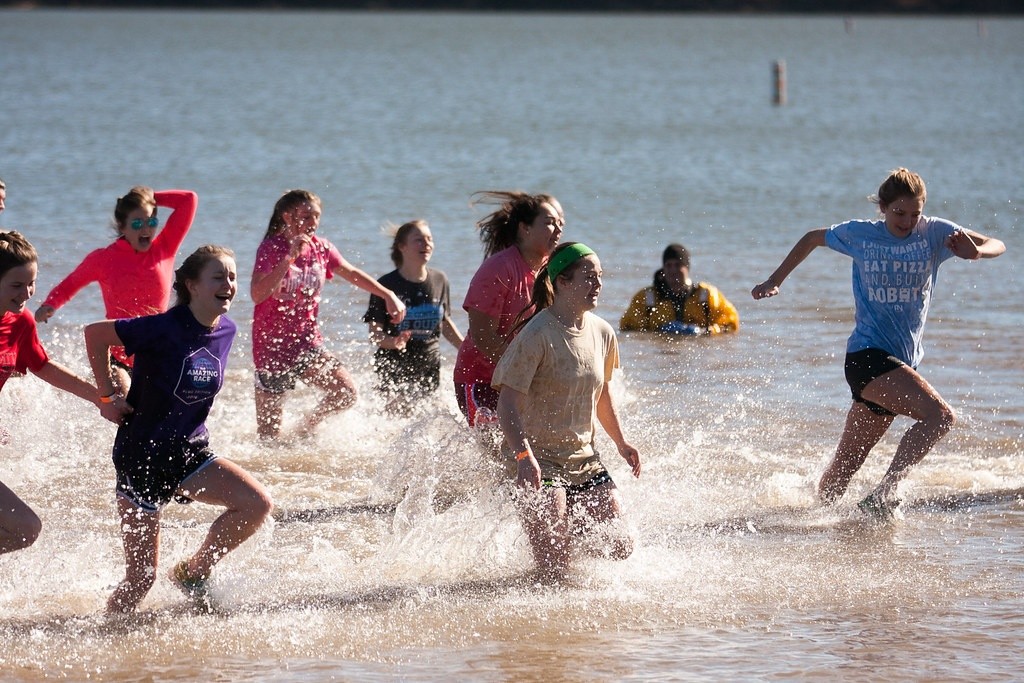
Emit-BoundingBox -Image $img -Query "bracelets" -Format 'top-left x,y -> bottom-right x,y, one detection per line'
516,450 -> 532,460
98,391 -> 119,403
285,254 -> 295,263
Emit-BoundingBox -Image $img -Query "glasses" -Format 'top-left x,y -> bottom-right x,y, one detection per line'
122,218 -> 159,230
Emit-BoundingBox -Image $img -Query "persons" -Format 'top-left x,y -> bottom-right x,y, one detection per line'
250,188 -> 407,440
0,229 -> 128,555
361,219 -> 465,417
453,191 -> 565,431
620,244 -> 739,336
490,242 -> 641,578
86,244 -> 274,614
751,167 -> 1007,522
34,185 -> 199,378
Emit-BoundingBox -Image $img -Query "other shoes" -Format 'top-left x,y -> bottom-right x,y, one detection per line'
167,562 -> 211,607
859,495 -> 903,526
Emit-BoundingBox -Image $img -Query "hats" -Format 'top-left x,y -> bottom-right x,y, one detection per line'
663,242 -> 690,269
